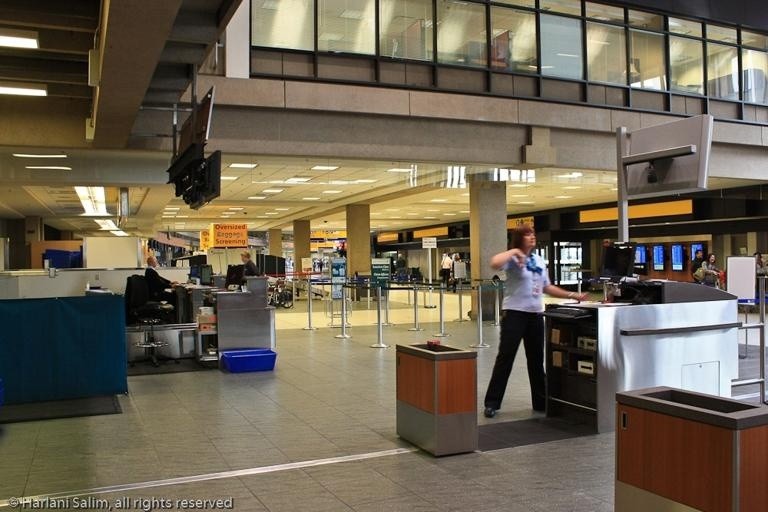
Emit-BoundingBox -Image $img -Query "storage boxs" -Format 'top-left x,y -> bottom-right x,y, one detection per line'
222,348 -> 277,373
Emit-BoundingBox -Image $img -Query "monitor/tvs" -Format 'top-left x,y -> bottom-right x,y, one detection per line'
190,150 -> 221,210
190,265 -> 213,284
225,264 -> 244,290
599,242 -> 637,277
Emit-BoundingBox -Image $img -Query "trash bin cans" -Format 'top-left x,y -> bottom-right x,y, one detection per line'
356,271 -> 373,301
471,279 -> 505,321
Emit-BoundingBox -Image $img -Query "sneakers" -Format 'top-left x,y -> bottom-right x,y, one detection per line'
484,407 -> 495,418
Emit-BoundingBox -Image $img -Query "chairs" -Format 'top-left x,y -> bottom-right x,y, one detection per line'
125,274 -> 179,366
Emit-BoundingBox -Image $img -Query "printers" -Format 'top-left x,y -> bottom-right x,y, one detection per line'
85,282 -> 114,297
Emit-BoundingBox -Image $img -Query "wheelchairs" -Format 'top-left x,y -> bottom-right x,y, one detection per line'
267,277 -> 293,309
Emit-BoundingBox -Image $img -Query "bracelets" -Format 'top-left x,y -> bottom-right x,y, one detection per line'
568,291 -> 576,298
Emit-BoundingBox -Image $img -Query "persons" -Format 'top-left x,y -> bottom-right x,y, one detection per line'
143,255 -> 181,323
452,255 -> 463,294
483,222 -> 591,417
240,250 -> 261,276
701,253 -> 723,287
395,253 -> 407,286
441,252 -> 453,291
752,251 -> 768,292
598,238 -> 618,276
317,259 -> 324,272
691,249 -> 704,284
